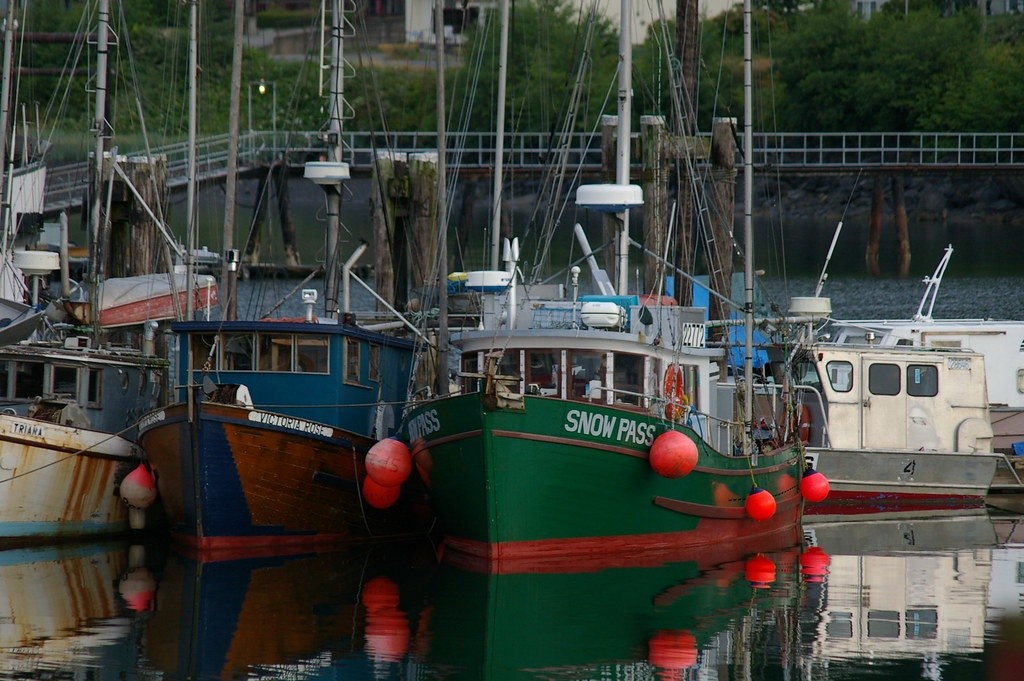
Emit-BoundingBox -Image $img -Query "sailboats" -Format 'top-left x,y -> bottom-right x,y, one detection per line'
1,0 -> 1024,558
1,516 -> 989,681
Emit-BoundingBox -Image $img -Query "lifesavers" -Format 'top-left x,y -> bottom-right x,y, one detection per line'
774,398 -> 813,450
257,310 -> 323,328
659,361 -> 685,421
